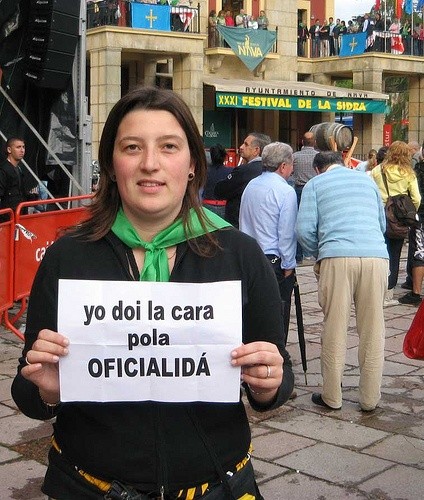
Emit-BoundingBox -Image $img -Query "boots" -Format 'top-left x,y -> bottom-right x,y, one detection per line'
384,288 -> 401,306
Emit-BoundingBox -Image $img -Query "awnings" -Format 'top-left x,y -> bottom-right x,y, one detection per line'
203,78 -> 390,116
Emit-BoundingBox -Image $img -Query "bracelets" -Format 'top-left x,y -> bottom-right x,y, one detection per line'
247,384 -> 267,395
39,392 -> 61,407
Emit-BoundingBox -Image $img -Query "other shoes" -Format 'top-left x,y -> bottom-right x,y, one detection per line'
304,256 -> 314,261
398,292 -> 422,305
401,282 -> 413,290
312,392 -> 342,411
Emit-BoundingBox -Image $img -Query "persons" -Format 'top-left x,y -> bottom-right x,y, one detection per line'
0,138 -> 39,223
10,88 -> 295,500
356,139 -> 424,306
296,149 -> 391,412
213,132 -> 270,230
86,1 -> 270,53
297,13 -> 424,58
202,143 -> 236,201
290,132 -> 321,265
239,141 -> 299,400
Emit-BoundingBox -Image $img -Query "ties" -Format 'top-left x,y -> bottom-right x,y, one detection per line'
111,204 -> 233,284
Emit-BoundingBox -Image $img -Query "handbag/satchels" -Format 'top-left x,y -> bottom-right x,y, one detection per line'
385,194 -> 421,240
402,297 -> 424,361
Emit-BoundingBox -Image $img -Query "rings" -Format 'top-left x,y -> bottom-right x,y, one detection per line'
265,364 -> 271,379
26,356 -> 32,365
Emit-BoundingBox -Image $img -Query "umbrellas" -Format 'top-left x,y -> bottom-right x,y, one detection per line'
294,276 -> 308,385
